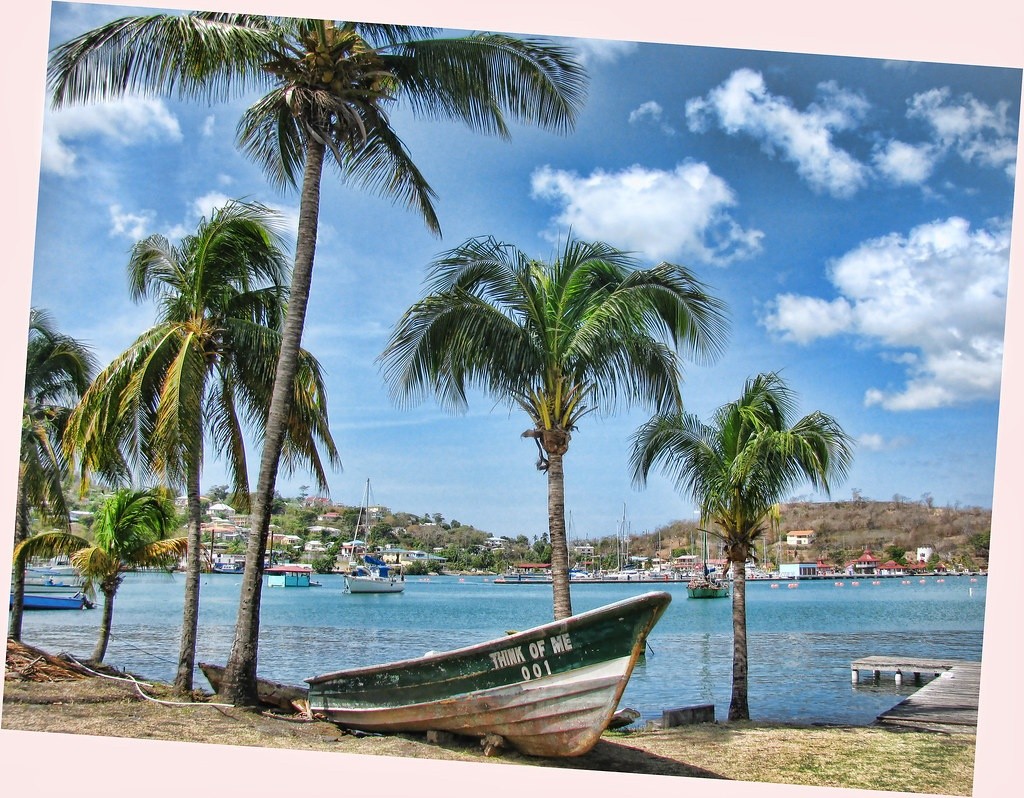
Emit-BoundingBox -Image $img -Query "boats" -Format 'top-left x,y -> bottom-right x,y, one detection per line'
213,562 -> 244,574
9,564 -> 95,610
303,590 -> 673,757
197,661 -> 310,714
121,564 -> 176,573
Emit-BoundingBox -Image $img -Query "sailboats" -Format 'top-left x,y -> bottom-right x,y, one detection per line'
343,477 -> 405,593
567,501 -> 715,580
686,529 -> 731,599
